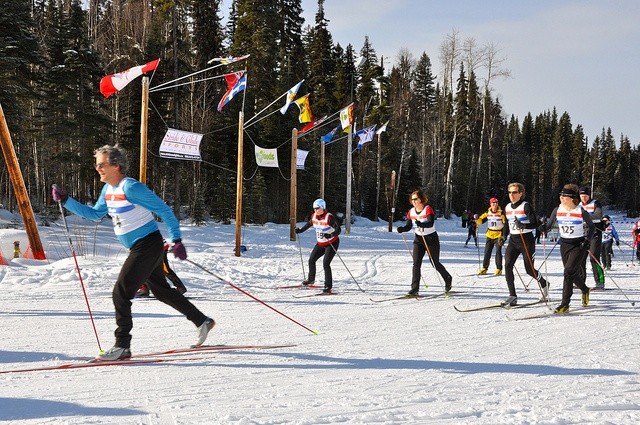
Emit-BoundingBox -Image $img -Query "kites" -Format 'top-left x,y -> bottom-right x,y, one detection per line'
294,92 -> 315,125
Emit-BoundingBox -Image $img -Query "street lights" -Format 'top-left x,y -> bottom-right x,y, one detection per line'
343,54 -> 367,232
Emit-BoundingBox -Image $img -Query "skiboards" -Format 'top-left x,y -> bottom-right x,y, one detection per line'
277,284 -> 338,298
0,344 -> 298,373
454,299 -> 552,312
505,305 -> 597,321
370,290 -> 463,302
456,273 -> 505,279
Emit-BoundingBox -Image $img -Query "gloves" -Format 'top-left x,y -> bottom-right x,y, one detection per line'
173,242 -> 187,260
397,227 -> 404,233
324,234 -> 332,238
581,239 -> 589,249
53,185 -> 66,202
498,236 -> 505,247
538,224 -> 545,233
415,221 -> 423,228
295,228 -> 301,234
514,220 -> 524,229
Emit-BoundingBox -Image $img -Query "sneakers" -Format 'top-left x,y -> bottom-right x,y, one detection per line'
496,269 -> 502,276
96,346 -> 131,361
501,296 -> 517,305
445,276 -> 452,292
409,290 -> 419,295
554,305 -> 569,314
176,286 -> 187,294
136,290 -> 149,297
302,279 -> 315,284
188,318 -> 215,348
539,280 -> 550,302
323,286 -> 332,292
478,268 -> 488,275
582,288 -> 590,307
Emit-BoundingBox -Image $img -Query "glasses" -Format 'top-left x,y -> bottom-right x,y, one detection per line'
411,197 -> 419,201
508,191 -> 518,194
493,202 -> 497,203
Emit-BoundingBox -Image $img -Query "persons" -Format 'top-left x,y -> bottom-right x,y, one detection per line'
51,143 -> 216,362
462,210 -> 468,228
473,197 -> 506,276
294,198 -> 342,294
631,219 -> 640,264
498,182 -> 550,306
464,217 -> 479,248
537,183 -> 595,314
135,234 -> 188,298
541,216 -> 548,238
397,190 -> 452,296
601,215 -> 620,270
467,210 -> 473,222
535,216 -> 542,244
577,187 -> 605,292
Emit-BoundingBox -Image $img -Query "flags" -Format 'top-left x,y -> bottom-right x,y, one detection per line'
291,117 -> 325,134
279,77 -> 304,116
206,53 -> 245,66
97,56 -> 161,99
343,124 -> 353,135
317,124 -> 342,142
216,70 -> 247,112
338,102 -> 355,129
376,117 -> 390,136
356,123 -> 376,150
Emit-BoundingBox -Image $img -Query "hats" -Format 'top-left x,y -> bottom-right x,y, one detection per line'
579,187 -> 590,196
559,188 -> 579,199
313,199 -> 326,210
489,198 -> 498,204
